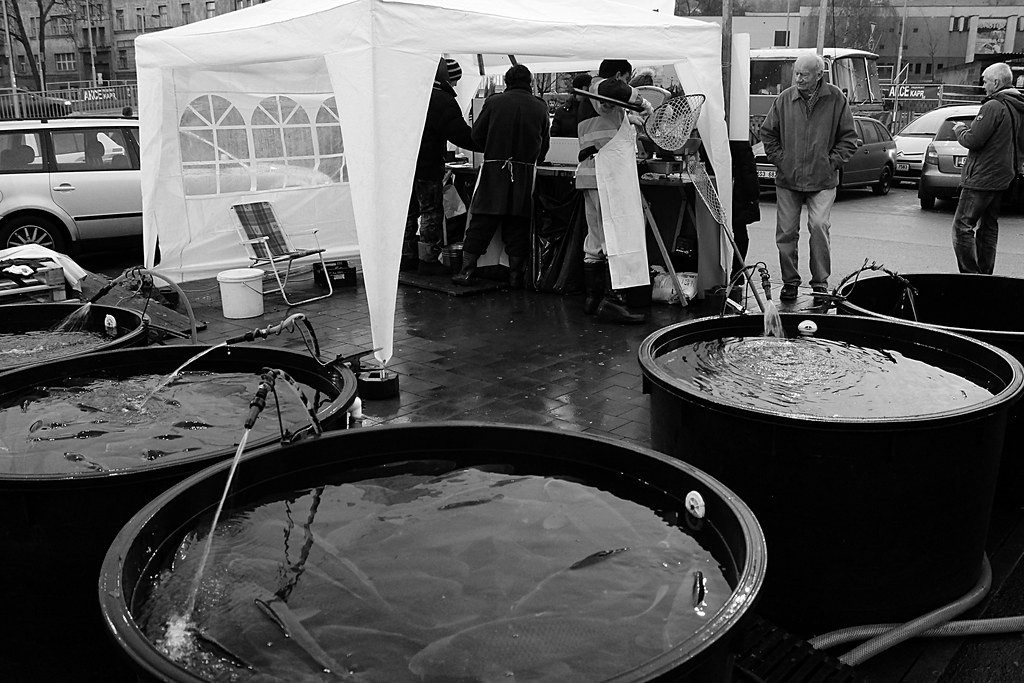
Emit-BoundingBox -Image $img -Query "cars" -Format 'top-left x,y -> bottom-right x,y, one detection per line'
0,103 -> 333,261
916,110 -> 980,212
0,87 -> 73,121
892,103 -> 984,188
753,114 -> 898,195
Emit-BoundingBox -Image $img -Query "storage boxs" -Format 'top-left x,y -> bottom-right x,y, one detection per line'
311,260 -> 356,291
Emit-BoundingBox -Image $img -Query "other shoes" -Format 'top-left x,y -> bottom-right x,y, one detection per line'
779,284 -> 798,299
812,287 -> 829,304
417,260 -> 449,276
400,255 -> 420,272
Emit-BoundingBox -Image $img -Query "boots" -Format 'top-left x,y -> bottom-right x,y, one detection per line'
450,251 -> 479,286
595,260 -> 646,325
581,263 -> 609,315
507,256 -> 525,291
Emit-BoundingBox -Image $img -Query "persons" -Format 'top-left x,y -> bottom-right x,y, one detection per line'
401,58 -> 485,276
698,139 -> 761,291
951,62 -> 1024,276
577,59 -> 649,323
550,72 -> 700,161
452,65 -> 551,289
760,54 -> 859,299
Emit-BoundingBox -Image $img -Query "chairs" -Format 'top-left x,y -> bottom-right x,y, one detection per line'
88,139 -> 104,168
111,153 -> 129,170
229,200 -> 333,307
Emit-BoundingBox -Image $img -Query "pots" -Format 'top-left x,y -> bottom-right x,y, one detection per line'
638,137 -> 702,155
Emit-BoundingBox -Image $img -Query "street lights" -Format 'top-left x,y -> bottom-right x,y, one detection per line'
140,5 -> 161,34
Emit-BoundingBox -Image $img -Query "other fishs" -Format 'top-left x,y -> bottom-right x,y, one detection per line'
141,463 -> 715,683
0,369 -> 303,475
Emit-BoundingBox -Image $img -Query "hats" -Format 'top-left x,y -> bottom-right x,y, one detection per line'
571,73 -> 593,88
445,58 -> 462,81
628,68 -> 656,88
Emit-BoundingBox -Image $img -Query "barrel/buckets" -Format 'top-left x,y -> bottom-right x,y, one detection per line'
836,273 -> 1024,369
637,313 -> 1024,670
0,303 -> 766,683
217,268 -> 265,318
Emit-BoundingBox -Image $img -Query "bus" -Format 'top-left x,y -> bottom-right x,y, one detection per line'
528,45 -> 891,141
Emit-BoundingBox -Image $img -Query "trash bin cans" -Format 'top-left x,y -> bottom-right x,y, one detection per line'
440,242 -> 463,277
216,268 -> 266,319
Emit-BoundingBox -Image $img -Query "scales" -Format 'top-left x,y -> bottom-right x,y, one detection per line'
637,136 -> 703,180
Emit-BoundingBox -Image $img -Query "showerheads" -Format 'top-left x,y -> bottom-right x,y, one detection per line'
226,331 -> 255,346
89,285 -> 112,303
761,281 -> 773,301
244,397 -> 266,430
897,275 -> 909,288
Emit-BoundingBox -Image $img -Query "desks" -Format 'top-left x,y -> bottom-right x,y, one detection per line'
440,169 -> 479,246
535,167 -> 716,306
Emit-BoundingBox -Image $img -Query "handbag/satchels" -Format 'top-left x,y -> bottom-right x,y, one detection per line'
1001,173 -> 1024,205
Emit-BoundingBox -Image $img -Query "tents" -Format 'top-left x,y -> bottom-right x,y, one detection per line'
133,0 -> 748,398
